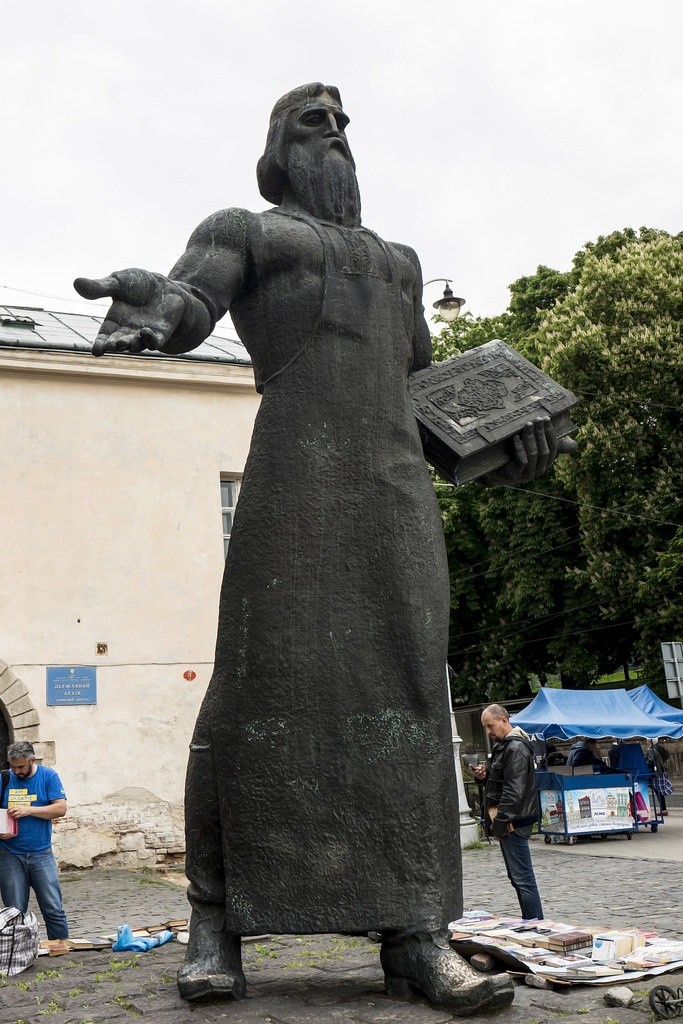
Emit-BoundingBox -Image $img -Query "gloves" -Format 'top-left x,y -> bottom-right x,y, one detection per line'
489,817 -> 509,837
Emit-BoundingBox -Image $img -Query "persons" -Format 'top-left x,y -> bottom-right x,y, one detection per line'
0,741 -> 67,942
539,744 -> 557,770
73,83 -> 516,1017
608,737 -> 620,767
462,742 -> 479,767
645,738 -> 674,816
471,704 -> 544,920
567,737 -> 607,767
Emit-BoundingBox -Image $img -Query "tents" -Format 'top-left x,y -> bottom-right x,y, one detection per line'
626,683 -> 683,725
509,687 -> 683,739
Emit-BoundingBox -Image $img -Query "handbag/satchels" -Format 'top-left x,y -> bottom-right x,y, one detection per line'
0,906 -> 40,977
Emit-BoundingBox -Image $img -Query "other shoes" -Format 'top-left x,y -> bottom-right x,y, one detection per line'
657,810 -> 668,816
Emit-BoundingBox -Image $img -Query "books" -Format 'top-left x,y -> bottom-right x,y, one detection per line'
39,919 -> 189,956
449,910 -> 683,980
407,339 -> 580,486
